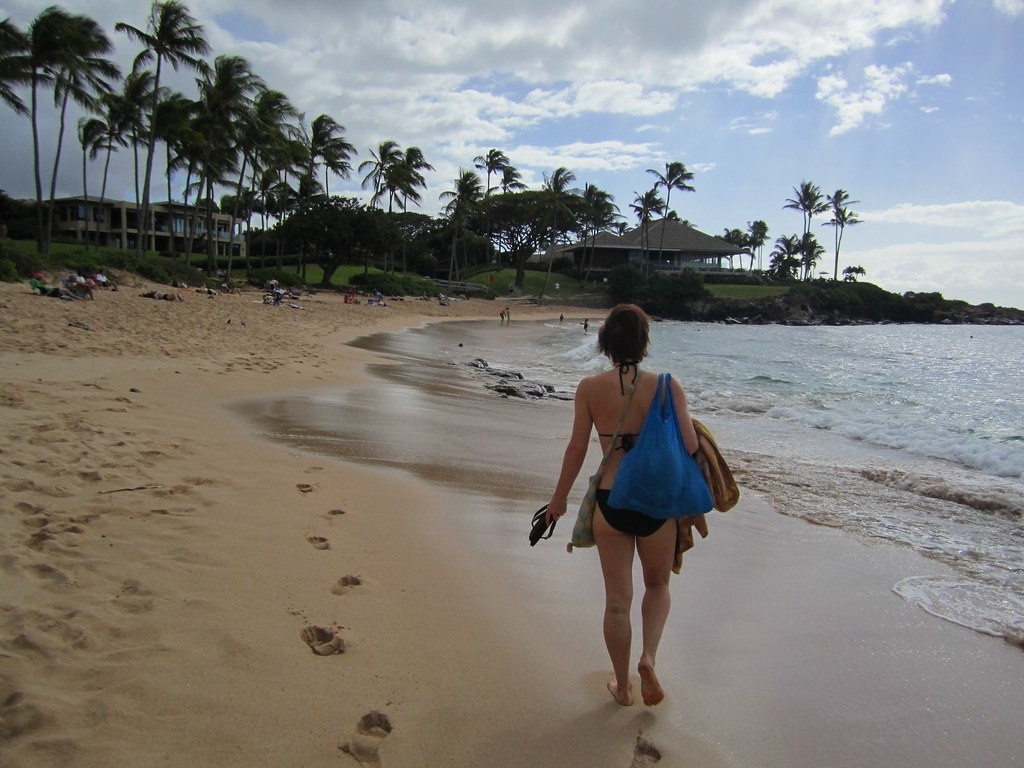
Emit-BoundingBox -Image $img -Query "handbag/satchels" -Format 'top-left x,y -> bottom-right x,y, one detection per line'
571,474 -> 596,548
605,371 -> 714,520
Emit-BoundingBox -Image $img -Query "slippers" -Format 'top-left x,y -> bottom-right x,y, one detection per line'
528,504 -> 558,547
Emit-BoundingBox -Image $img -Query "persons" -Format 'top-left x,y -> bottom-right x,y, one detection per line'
546,306 -> 700,708
29,271 -> 591,335
